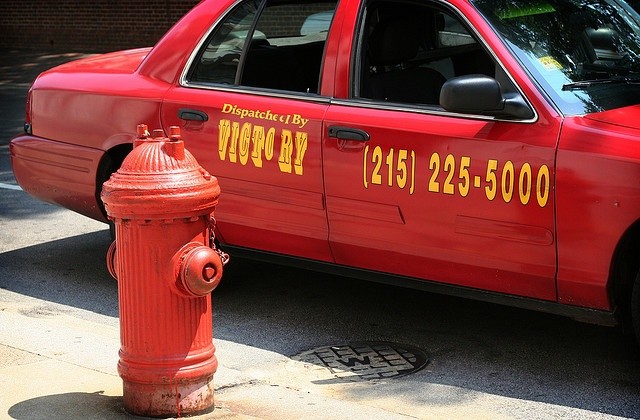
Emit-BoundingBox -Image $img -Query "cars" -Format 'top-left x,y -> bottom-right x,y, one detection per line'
10,0 -> 640,327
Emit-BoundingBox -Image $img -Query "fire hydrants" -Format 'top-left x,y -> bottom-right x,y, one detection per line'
100,124 -> 230,417
